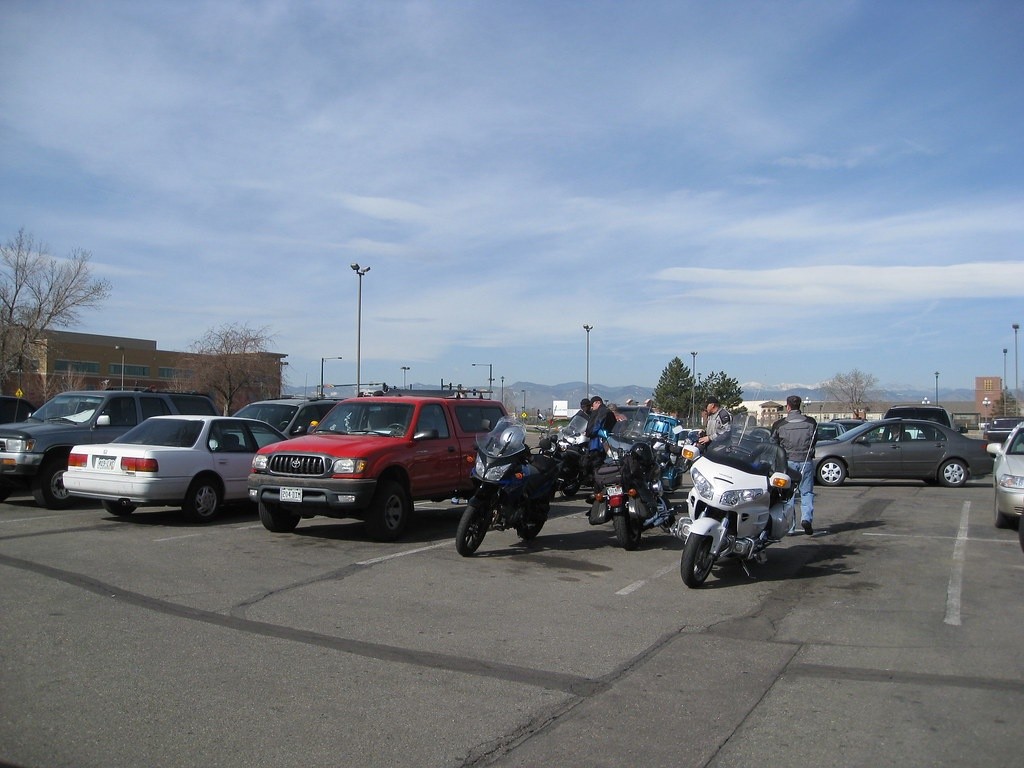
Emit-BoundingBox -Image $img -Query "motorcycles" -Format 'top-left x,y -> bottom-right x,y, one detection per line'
531,402 -> 702,550
454,416 -> 588,557
674,427 -> 804,588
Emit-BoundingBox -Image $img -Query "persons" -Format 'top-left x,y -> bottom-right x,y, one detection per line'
698,396 -> 733,453
570,395 -> 628,490
769,396 -> 819,536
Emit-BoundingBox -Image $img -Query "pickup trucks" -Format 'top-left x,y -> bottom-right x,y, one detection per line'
246,389 -> 511,543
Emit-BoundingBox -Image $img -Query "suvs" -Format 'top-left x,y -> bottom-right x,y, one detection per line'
231,396 -> 344,451
0,384 -> 224,510
830,405 -> 956,441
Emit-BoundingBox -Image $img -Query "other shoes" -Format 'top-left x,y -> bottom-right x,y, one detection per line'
801,520 -> 813,535
788,533 -> 794,536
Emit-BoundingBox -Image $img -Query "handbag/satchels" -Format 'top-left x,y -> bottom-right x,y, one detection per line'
594,466 -> 621,486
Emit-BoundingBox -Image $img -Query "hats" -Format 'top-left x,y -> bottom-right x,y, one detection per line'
702,396 -> 718,406
587,396 -> 602,405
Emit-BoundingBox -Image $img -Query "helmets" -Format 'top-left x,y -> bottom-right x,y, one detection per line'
500,427 -> 524,448
631,443 -> 652,460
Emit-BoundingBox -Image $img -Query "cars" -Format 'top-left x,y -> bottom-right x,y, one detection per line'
0,396 -> 38,424
984,416 -> 1024,527
62,416 -> 292,524
811,417 -> 995,487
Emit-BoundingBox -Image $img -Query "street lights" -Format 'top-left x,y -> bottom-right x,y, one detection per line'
1002,349 -> 1008,416
500,376 -> 505,402
804,396 -> 812,415
321,357 -> 342,399
1011,323 -> 1019,417
400,366 -> 410,388
116,346 -> 125,389
934,372 -> 940,405
351,264 -> 371,396
691,351 -> 698,428
472,363 -> 492,399
582,324 -> 595,397
982,397 -> 991,430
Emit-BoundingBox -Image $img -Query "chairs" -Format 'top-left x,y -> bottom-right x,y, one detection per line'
904,432 -> 911,440
367,412 -> 387,429
222,434 -> 239,448
